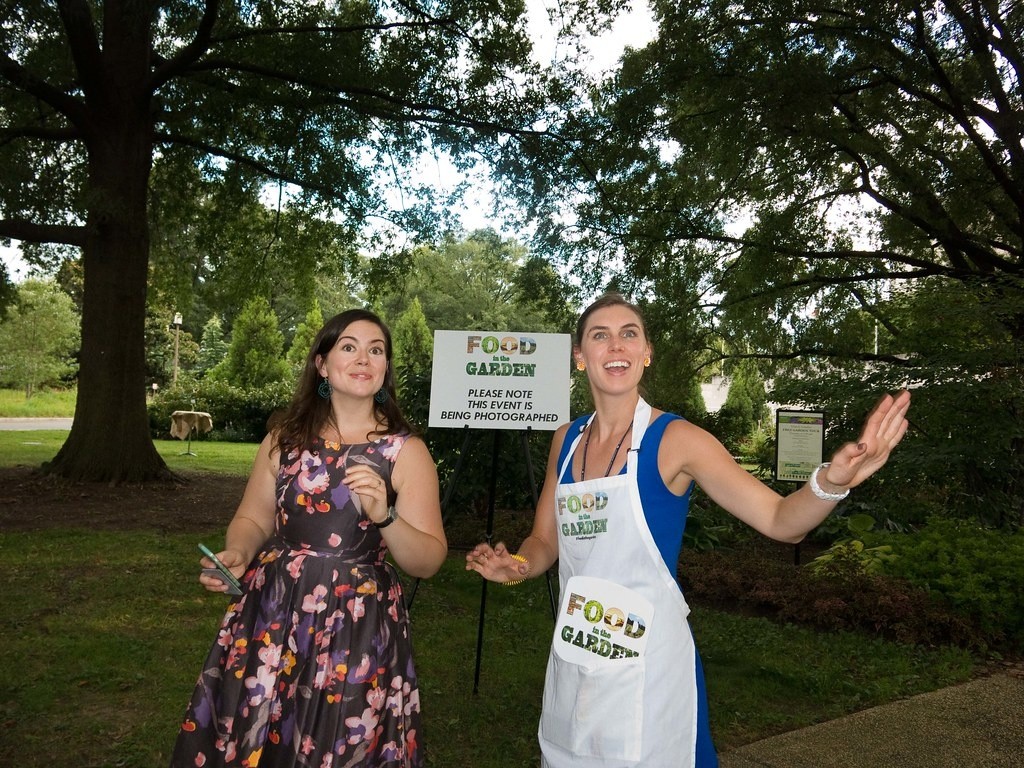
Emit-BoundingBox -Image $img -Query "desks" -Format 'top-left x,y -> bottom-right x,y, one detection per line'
169,410 -> 213,458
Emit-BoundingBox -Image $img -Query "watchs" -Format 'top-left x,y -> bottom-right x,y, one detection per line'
372,506 -> 398,528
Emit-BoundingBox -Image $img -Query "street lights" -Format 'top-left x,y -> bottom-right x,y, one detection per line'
172,312 -> 183,389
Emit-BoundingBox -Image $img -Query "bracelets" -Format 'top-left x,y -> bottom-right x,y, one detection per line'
810,462 -> 850,501
503,555 -> 532,586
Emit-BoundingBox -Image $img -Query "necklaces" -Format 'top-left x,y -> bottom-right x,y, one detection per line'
581,423 -> 632,481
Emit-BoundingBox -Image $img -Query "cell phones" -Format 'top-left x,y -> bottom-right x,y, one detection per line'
197,543 -> 241,588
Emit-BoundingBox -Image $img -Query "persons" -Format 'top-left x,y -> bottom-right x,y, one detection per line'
171,309 -> 448,768
466,294 -> 911,768
